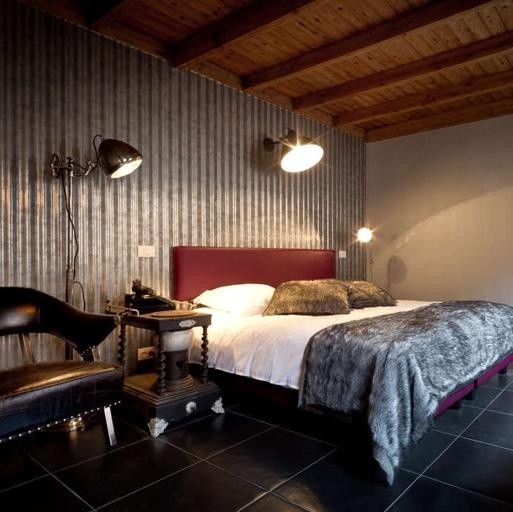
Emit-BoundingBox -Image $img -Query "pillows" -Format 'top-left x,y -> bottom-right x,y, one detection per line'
344,277 -> 399,311
262,277 -> 350,320
192,283 -> 276,316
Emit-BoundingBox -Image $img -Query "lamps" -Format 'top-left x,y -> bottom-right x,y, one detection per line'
48,133 -> 143,433
261,129 -> 325,176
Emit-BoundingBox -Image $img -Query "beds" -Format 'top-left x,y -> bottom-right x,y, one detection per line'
171,245 -> 513,486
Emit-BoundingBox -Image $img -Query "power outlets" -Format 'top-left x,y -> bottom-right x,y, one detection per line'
136,347 -> 156,361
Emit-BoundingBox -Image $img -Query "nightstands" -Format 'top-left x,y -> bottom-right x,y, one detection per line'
105,306 -> 226,439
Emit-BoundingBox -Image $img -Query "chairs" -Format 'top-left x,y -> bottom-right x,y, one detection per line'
0,285 -> 126,451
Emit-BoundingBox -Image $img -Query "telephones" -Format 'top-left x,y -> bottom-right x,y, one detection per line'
129,295 -> 175,315
